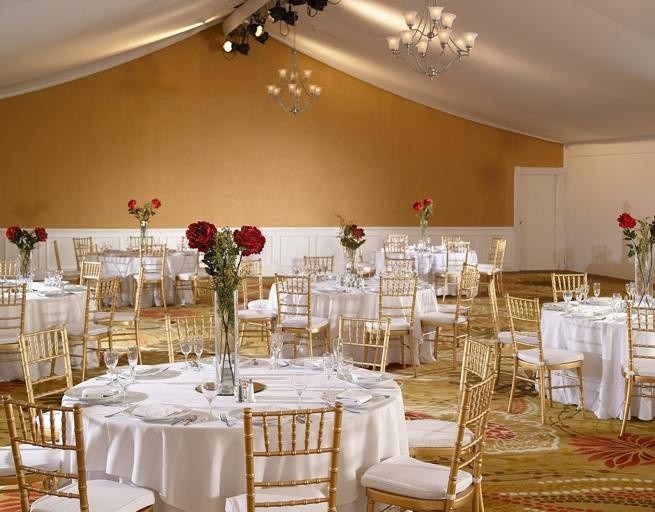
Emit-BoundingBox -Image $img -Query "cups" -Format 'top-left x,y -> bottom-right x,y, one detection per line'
97,240 -> 112,254
387,240 -> 465,254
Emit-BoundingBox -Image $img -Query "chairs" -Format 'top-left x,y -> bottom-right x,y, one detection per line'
1,235 -> 511,381
222,400 -> 346,511
360,368 -> 500,512
14,322 -> 76,443
501,293 -> 588,425
618,302 -> 655,437
485,279 -> 539,386
405,334 -> 495,464
2,394 -> 156,512
550,269 -> 589,301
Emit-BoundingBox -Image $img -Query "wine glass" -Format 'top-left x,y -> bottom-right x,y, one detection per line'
42,269 -> 65,291
559,282 -> 640,321
101,334 -> 354,426
290,261 -> 419,294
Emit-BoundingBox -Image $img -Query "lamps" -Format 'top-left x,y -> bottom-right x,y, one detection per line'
265,5 -> 322,118
221,1 -> 341,60
385,0 -> 479,78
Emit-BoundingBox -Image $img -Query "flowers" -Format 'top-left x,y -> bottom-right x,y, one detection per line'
4,225 -> 48,279
126,199 -> 162,244
616,210 -> 655,309
186,221 -> 266,386
334,214 -> 367,277
412,198 -> 434,227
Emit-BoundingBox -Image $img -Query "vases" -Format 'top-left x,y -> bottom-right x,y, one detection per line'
419,219 -> 430,252
137,220 -> 149,252
631,241 -> 655,309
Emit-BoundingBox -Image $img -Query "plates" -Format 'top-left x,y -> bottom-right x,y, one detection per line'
344,370 -> 395,388
320,387 -> 394,407
81,385 -> 123,404
